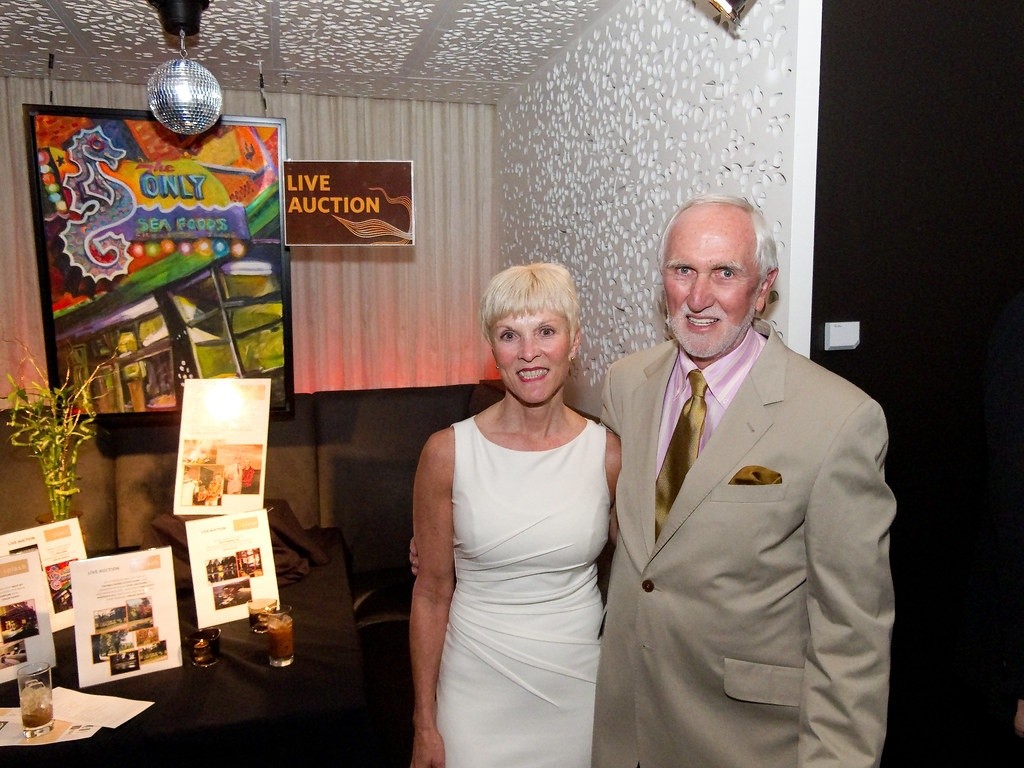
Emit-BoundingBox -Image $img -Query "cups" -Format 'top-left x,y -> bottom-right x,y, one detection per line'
17,663 -> 55,738
267,606 -> 295,667
247,599 -> 277,633
185,629 -> 221,666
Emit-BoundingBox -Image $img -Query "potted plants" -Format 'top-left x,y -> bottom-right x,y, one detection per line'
0,339 -> 124,559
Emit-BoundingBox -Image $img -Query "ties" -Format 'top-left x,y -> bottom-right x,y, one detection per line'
654,369 -> 708,545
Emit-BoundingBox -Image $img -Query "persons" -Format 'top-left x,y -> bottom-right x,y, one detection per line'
410,262 -> 620,768
410,195 -> 896,768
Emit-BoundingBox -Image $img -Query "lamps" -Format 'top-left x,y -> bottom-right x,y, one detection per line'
148,0 -> 223,135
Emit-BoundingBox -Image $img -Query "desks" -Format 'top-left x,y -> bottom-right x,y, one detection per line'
0,528 -> 370,768
